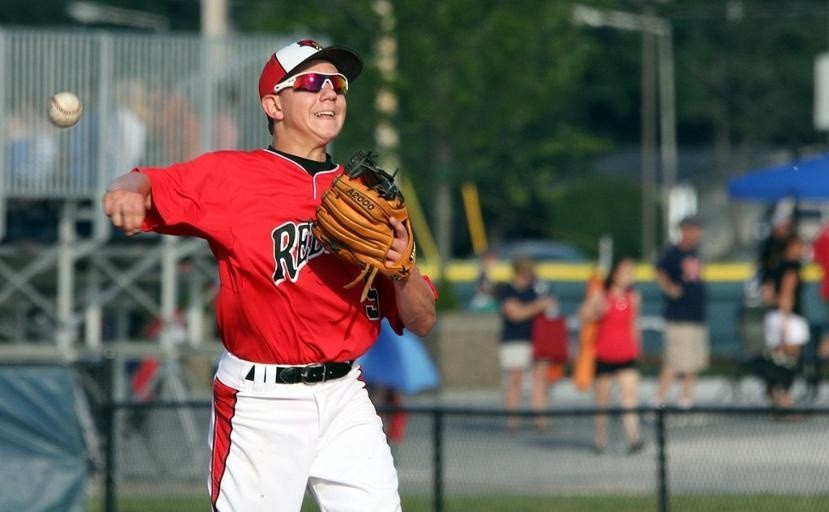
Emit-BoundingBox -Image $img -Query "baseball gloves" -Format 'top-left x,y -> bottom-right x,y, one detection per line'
312,153 -> 418,304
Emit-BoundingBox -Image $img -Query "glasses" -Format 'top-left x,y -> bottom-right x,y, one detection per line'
273,70 -> 350,97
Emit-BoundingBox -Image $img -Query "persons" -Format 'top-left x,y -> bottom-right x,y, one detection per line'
101,41 -> 438,512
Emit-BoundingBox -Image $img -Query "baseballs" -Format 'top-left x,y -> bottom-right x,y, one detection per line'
48,93 -> 83,128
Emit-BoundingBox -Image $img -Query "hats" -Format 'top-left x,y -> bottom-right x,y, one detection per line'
258,39 -> 364,100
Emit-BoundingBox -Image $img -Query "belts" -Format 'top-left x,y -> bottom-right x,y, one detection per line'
245,359 -> 355,387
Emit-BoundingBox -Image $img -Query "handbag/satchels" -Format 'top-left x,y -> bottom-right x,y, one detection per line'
530,306 -> 569,363
